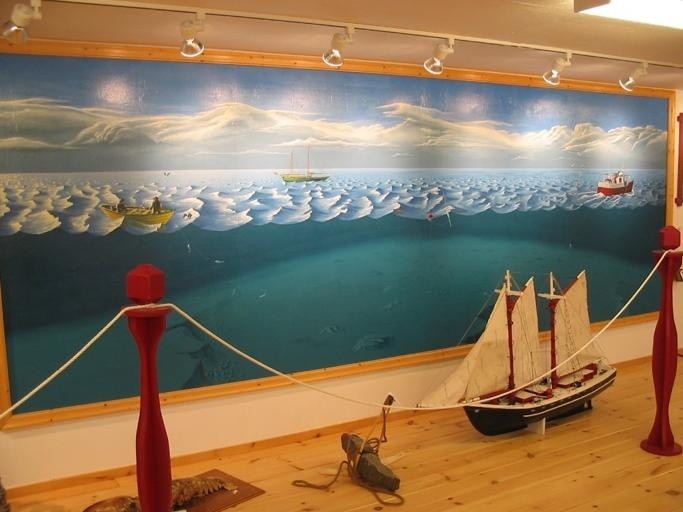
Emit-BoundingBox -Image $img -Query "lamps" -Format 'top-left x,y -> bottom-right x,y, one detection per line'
0,1 -> 650,95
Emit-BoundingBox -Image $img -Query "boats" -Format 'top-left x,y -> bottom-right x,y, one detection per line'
596,170 -> 634,196
99,196 -> 174,225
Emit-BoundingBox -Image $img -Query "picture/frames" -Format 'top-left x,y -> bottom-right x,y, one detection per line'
0,35 -> 677,430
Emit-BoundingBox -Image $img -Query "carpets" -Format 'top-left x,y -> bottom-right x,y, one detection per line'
81,467 -> 266,512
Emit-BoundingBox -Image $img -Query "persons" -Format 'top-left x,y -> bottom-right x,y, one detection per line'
149,197 -> 161,214
116,199 -> 127,212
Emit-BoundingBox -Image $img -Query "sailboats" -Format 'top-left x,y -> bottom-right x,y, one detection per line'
274,146 -> 330,183
413,269 -> 616,435
